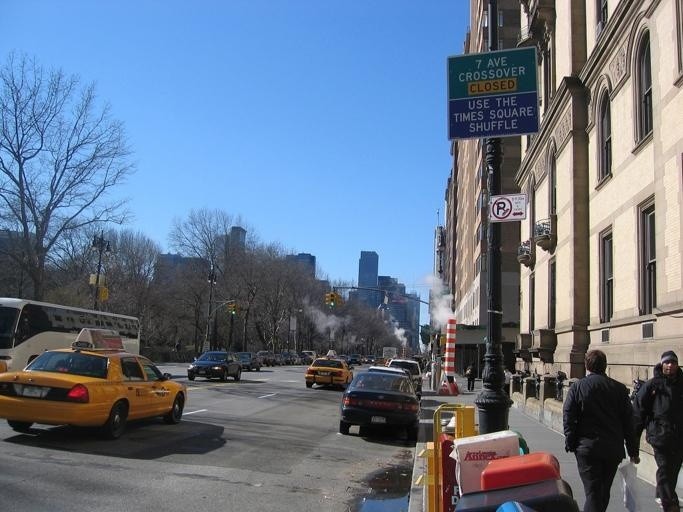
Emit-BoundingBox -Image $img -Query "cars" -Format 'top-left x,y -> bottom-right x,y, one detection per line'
306,349 -> 354,392
339,354 -> 423,441
237,350 -> 316,371
0,328 -> 187,440
187,351 -> 242,382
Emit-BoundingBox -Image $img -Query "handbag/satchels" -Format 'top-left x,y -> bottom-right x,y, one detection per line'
620,463 -> 637,511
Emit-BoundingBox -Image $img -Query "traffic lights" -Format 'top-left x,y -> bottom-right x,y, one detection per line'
232,304 -> 236,315
328,292 -> 335,309
335,291 -> 341,306
237,304 -> 240,315
228,304 -> 232,314
324,293 -> 329,306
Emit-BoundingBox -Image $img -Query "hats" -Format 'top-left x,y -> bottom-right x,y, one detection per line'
661,351 -> 678,365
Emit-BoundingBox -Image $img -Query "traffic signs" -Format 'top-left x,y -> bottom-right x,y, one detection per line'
445,45 -> 540,137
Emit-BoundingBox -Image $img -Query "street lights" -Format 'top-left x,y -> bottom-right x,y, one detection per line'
89,230 -> 111,312
288,308 -> 303,352
205,262 -> 217,351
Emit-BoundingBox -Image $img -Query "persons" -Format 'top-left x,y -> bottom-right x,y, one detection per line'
466,361 -> 475,392
562,349 -> 639,512
504,367 -> 512,395
633,351 -> 682,512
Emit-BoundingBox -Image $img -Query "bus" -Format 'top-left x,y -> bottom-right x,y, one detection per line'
0,296 -> 141,373
